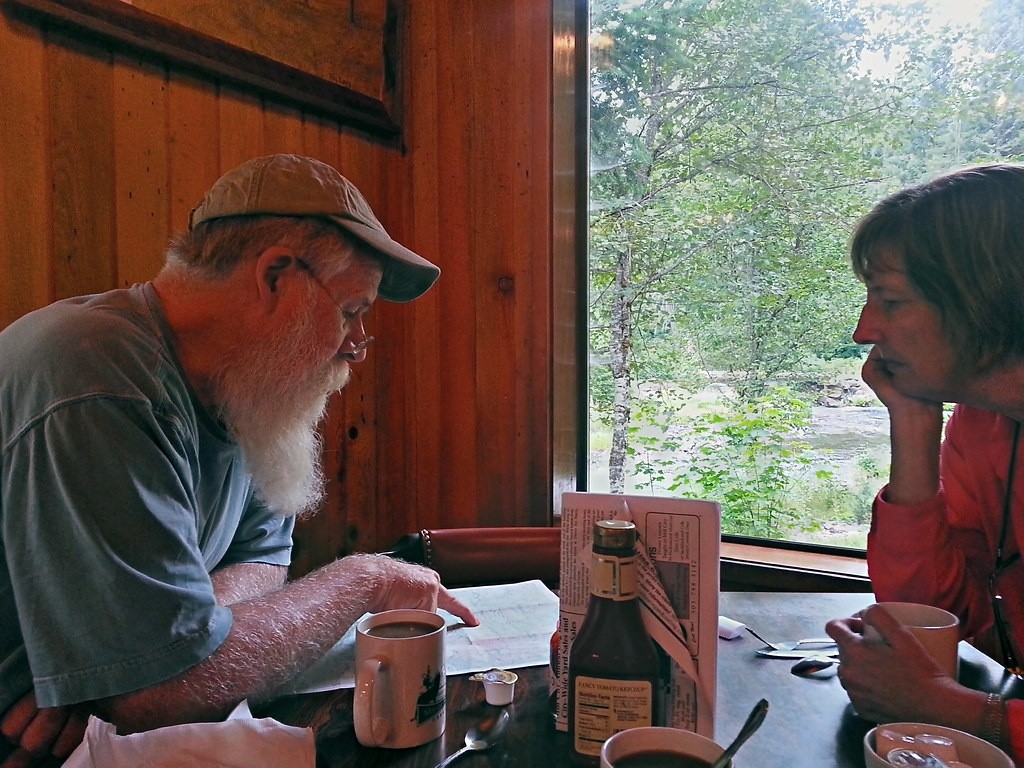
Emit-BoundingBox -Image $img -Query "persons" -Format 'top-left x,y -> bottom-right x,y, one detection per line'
0,151 -> 481,768
822,160 -> 1024,768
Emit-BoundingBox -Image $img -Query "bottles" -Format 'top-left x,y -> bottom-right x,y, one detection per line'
568,520 -> 667,767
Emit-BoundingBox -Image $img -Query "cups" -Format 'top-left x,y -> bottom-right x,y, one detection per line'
354,609 -> 446,748
851,602 -> 961,685
601,726 -> 736,768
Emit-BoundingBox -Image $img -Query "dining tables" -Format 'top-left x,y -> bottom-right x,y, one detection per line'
32,587 -> 1024,767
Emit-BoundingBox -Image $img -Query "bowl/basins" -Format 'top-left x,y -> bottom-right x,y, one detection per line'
863,722 -> 1015,768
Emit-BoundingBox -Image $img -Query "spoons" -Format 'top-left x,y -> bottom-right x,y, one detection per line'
790,655 -> 843,675
712,698 -> 769,768
434,707 -> 510,768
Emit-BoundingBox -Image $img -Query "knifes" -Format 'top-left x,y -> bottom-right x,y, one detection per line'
755,649 -> 841,659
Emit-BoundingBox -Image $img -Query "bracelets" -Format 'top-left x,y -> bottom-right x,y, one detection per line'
980,690 -> 1004,753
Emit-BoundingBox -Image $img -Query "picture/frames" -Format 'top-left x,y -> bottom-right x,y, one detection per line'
7,0 -> 405,143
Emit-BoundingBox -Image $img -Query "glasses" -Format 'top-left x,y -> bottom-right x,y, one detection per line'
295,257 -> 376,354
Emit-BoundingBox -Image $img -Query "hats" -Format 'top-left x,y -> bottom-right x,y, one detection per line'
187,154 -> 440,304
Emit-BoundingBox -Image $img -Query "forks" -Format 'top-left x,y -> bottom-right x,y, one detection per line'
744,627 -> 838,652
